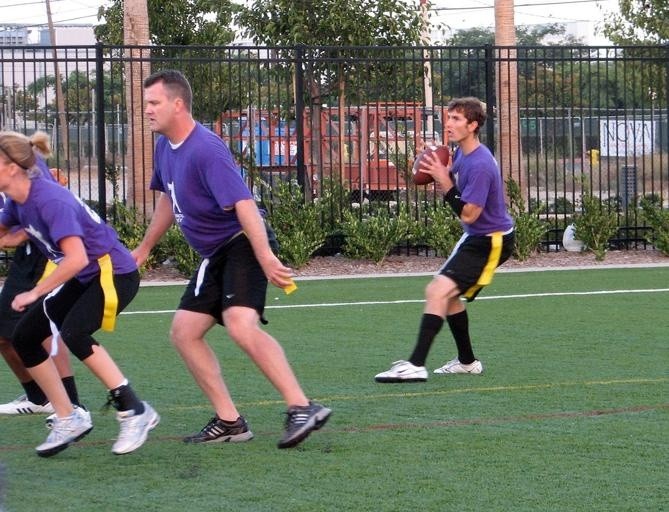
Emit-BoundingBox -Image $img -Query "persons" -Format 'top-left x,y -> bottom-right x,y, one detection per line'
0,151 -> 89,423
375,95 -> 517,384
0,128 -> 161,459
130,68 -> 333,449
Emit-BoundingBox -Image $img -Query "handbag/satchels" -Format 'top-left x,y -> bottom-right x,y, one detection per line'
563,223 -> 583,252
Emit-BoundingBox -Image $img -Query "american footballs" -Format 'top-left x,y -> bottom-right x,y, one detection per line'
411,146 -> 449,184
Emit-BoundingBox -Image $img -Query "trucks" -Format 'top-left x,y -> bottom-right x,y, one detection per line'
215,104 -> 454,195
368,131 -> 439,156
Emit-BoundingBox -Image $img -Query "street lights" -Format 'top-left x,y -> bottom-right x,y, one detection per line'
647,85 -> 658,119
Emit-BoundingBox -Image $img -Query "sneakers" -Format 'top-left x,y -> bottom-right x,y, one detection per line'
434,356 -> 482,374
46,403 -> 92,429
183,413 -> 254,443
35,411 -> 94,456
375,360 -> 429,382
111,401 -> 160,454
0,394 -> 55,415
278,397 -> 332,449
